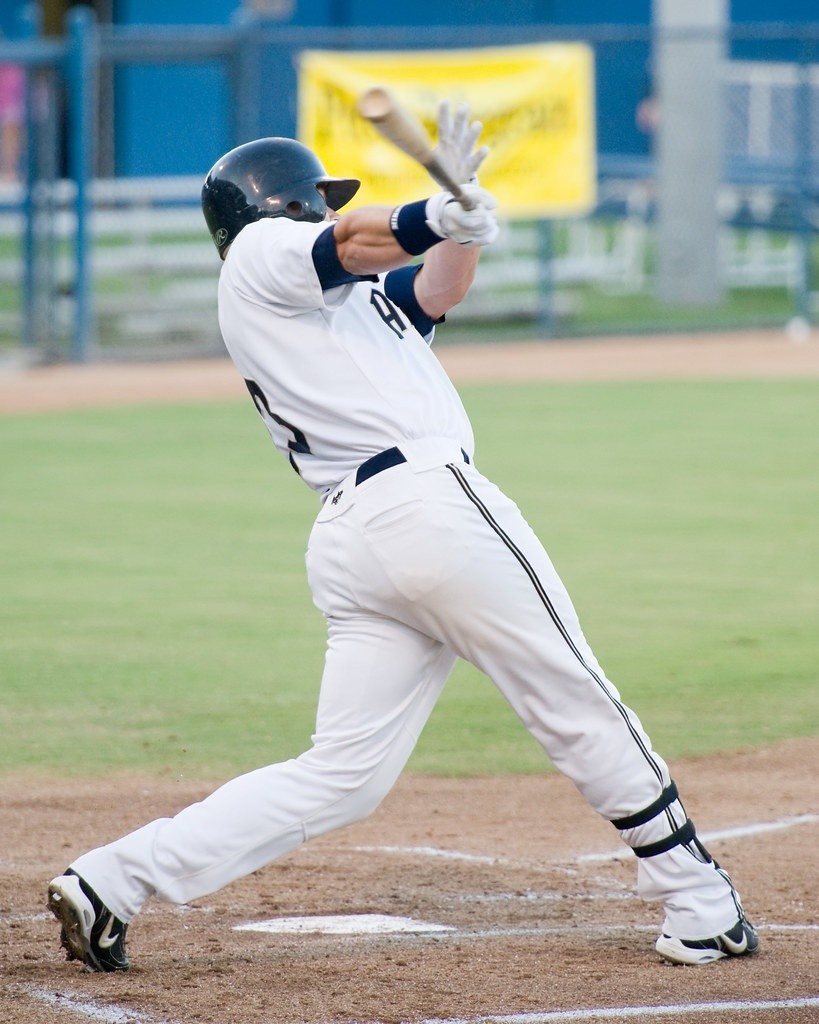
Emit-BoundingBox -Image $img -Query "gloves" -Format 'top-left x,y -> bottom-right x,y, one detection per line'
431,99 -> 490,188
424,185 -> 500,247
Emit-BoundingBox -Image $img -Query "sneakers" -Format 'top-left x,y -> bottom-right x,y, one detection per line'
46,866 -> 131,974
655,915 -> 761,965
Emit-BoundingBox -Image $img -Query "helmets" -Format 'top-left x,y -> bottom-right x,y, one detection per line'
201,137 -> 361,261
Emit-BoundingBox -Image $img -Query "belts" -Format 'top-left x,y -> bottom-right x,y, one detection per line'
356,443 -> 470,486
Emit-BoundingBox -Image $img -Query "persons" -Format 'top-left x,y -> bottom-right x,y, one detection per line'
42,104 -> 760,973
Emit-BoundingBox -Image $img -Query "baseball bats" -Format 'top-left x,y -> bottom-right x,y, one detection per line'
352,81 -> 476,212
